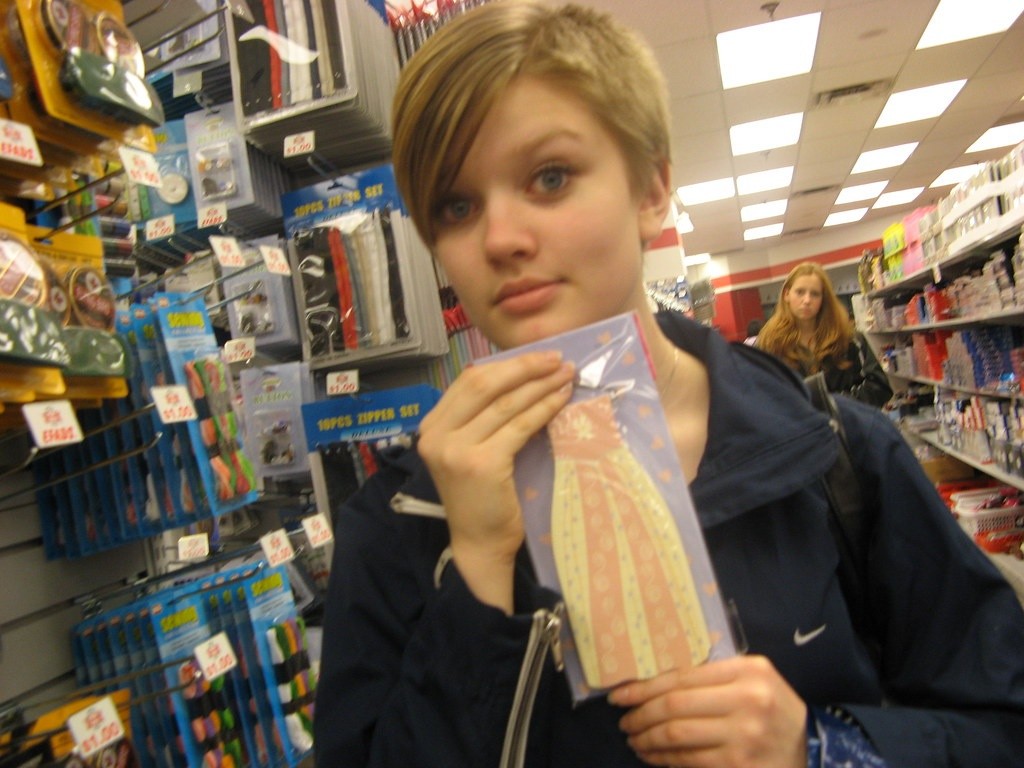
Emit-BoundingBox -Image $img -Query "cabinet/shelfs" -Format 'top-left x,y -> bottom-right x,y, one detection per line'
866,214 -> 1024,610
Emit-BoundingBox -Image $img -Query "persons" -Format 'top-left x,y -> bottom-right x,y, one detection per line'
743,261 -> 894,411
312,0 -> 1024,768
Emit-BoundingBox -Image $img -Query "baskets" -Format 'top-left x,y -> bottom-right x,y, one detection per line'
915,448 -> 1023,551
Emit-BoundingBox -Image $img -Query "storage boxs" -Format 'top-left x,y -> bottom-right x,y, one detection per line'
919,458 -> 972,486
951,486 -> 1024,535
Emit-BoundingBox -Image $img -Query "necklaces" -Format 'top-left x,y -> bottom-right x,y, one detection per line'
659,345 -> 679,401
